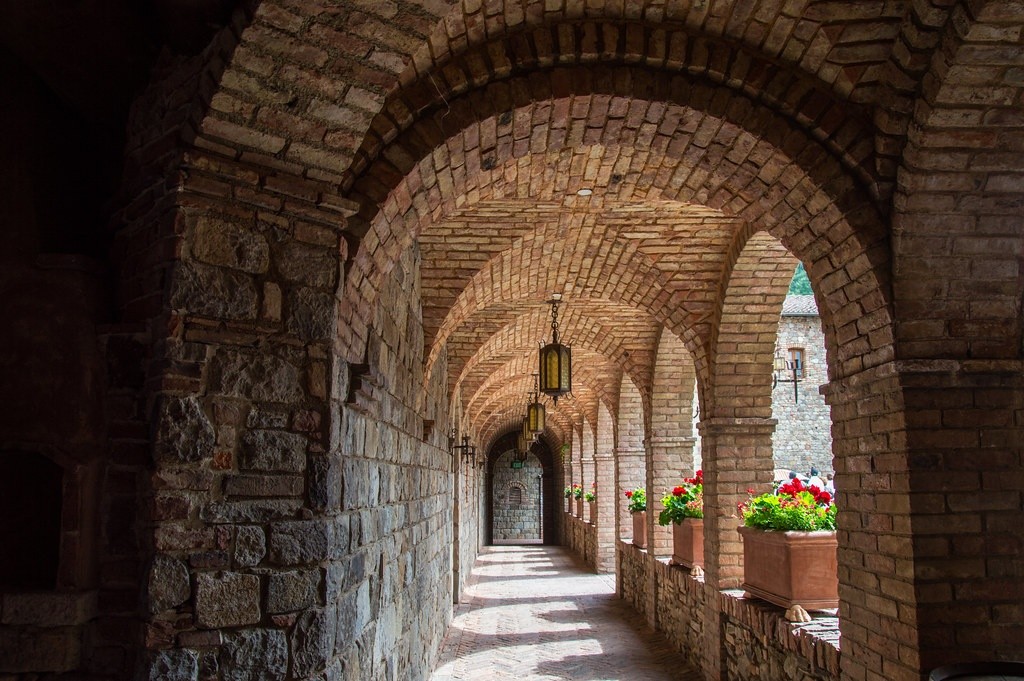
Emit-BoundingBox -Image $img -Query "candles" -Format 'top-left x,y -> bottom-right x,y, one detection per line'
464,432 -> 467,437
472,442 -> 476,446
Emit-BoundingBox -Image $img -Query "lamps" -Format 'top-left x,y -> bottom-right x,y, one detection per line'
517,373 -> 545,462
773,345 -> 786,378
536,298 -> 576,405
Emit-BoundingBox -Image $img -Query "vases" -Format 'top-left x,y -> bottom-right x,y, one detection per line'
589,501 -> 596,525
737,525 -> 839,622
575,497 -> 582,519
568,496 -> 572,514
669,517 -> 704,575
630,510 -> 648,548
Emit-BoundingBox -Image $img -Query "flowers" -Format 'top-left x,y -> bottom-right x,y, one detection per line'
586,482 -> 596,503
625,488 -> 648,513
563,485 -> 571,499
658,470 -> 704,526
730,476 -> 837,532
574,484 -> 581,501
